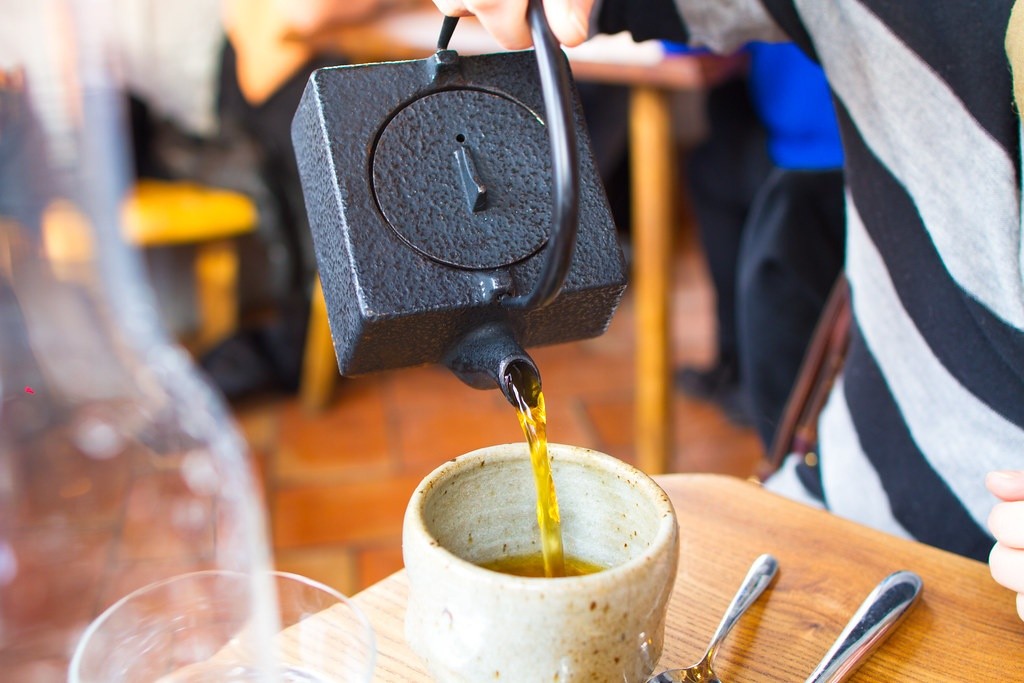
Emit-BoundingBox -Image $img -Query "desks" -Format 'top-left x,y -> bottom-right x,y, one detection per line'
283,7 -> 744,475
156,473 -> 1024,683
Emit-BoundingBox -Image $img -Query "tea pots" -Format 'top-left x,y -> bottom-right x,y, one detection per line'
290,1 -> 625,406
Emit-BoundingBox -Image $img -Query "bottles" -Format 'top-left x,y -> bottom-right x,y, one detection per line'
0,0 -> 284,683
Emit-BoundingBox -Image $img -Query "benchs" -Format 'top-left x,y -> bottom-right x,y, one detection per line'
0,178 -> 258,381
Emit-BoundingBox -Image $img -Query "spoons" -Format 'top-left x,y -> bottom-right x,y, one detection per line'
645,553 -> 779,682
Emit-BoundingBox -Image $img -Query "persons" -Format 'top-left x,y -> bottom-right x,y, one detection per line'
0,0 -> 1024,626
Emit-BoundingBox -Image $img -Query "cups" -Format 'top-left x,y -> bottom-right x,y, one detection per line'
400,440 -> 680,683
67,569 -> 378,683
